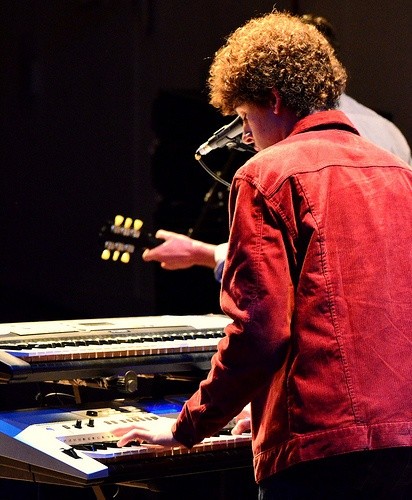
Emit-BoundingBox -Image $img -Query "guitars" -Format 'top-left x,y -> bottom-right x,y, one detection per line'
99,215 -> 166,263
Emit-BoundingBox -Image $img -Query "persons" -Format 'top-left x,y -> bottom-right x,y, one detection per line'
144,17 -> 412,281
112,13 -> 412,500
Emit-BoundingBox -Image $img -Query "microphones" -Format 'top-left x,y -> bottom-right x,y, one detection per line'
196,115 -> 242,155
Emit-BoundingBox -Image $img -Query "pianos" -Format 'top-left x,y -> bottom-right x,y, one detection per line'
0,394 -> 254,488
0,314 -> 235,385
